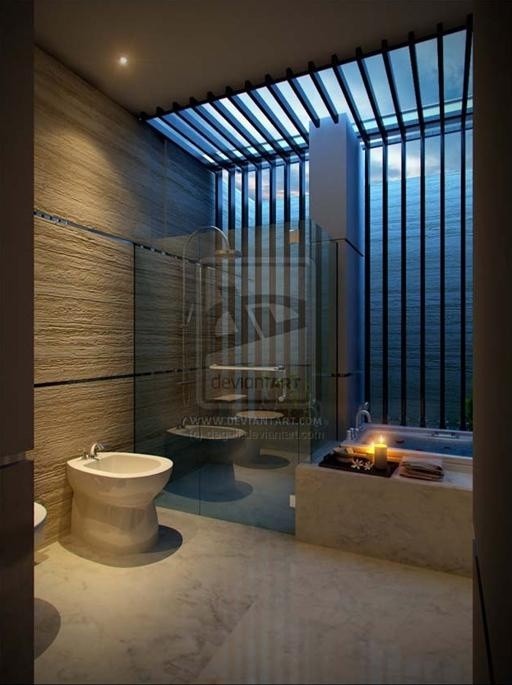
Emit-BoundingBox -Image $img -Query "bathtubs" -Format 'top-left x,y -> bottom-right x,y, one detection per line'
341,424 -> 473,465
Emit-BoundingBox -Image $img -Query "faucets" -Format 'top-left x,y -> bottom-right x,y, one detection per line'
179,416 -> 194,428
355,408 -> 371,433
89,442 -> 104,457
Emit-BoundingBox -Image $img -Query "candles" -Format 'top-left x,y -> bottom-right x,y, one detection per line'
373,434 -> 387,468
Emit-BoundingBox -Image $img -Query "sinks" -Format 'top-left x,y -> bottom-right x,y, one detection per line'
167,416 -> 247,493
66,452 -> 174,552
35,505 -> 45,530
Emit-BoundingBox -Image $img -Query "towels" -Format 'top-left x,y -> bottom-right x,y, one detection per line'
398,456 -> 444,481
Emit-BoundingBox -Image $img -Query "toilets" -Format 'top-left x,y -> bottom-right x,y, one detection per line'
213,394 -> 284,462
